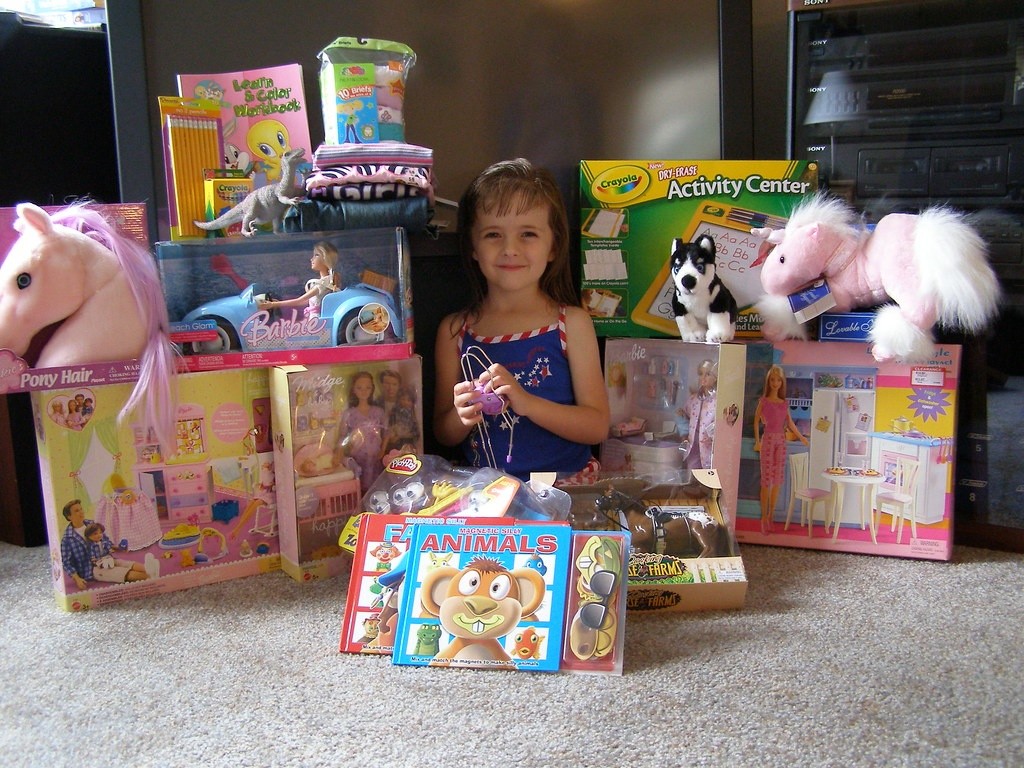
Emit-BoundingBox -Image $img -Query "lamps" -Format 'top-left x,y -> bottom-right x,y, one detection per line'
806,69 -> 866,180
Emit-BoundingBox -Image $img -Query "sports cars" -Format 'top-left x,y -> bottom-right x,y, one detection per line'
180,280 -> 404,357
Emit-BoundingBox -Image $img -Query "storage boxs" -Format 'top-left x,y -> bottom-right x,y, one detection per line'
268,358 -> 423,583
557,470 -> 747,614
29,370 -> 283,613
204,176 -> 256,237
580,161 -> 818,336
735,341 -> 964,561
155,230 -> 418,354
820,313 -> 876,344
600,340 -> 747,556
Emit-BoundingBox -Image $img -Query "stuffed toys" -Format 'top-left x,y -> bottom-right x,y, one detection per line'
751,193 -> 1002,359
0,196 -> 177,458
670,235 -> 737,343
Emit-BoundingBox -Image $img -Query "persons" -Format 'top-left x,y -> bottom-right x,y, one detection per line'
433,159 -> 611,485
257,241 -> 340,319
684,359 -> 718,469
338,372 -> 420,492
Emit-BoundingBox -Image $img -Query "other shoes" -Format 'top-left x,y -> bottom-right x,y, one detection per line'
145,554 -> 160,582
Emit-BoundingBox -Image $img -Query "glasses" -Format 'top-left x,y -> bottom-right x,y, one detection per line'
581,570 -> 616,630
